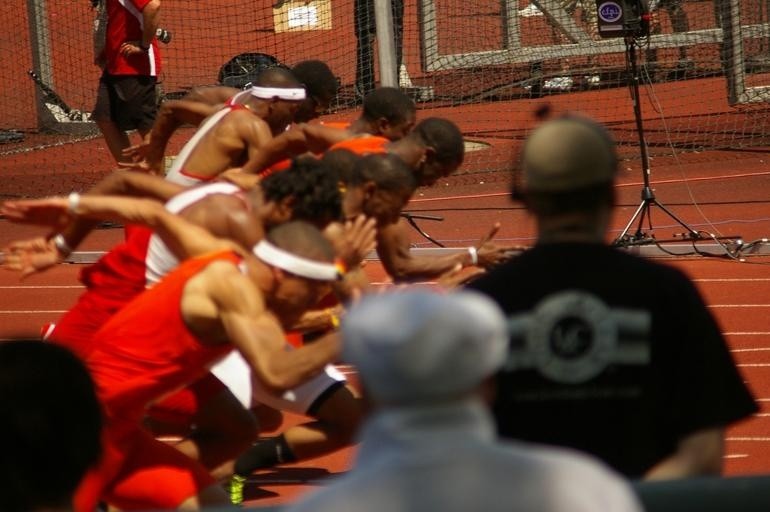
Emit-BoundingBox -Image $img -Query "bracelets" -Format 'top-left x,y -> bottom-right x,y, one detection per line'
138,40 -> 149,53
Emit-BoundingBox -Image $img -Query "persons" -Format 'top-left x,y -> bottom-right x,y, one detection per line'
87,0 -> 167,170
1,59 -> 526,510
459,114 -> 762,484
257,290 -> 646,512
644,0 -> 694,70
542,0 -> 600,89
353,0 -> 404,106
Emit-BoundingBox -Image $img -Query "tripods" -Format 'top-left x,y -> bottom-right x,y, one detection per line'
611,44 -> 700,245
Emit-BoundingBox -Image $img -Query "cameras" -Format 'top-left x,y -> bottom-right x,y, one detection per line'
155,28 -> 171,43
